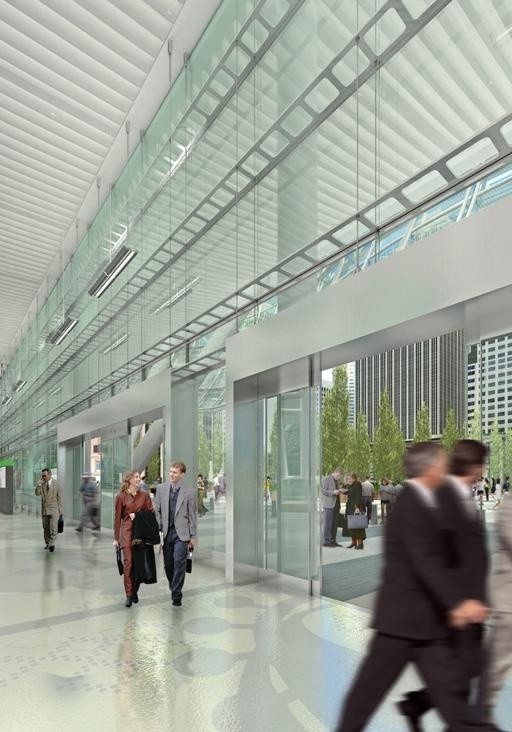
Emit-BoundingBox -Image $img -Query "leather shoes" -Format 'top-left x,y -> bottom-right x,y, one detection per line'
126,596 -> 133,606
133,593 -> 139,603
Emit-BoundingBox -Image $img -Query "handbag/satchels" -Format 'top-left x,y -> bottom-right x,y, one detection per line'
58,514 -> 64,534
347,512 -> 369,531
116,544 -> 123,577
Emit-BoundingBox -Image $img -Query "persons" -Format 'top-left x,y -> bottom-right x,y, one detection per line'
334,440 -> 503,731
155,462 -> 199,607
112,470 -> 155,609
197,474 -> 207,517
75,473 -> 100,533
320,466 -> 405,549
35,468 -> 64,552
472,476 -> 511,501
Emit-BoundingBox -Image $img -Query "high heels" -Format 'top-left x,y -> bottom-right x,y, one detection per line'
347,544 -> 364,549
